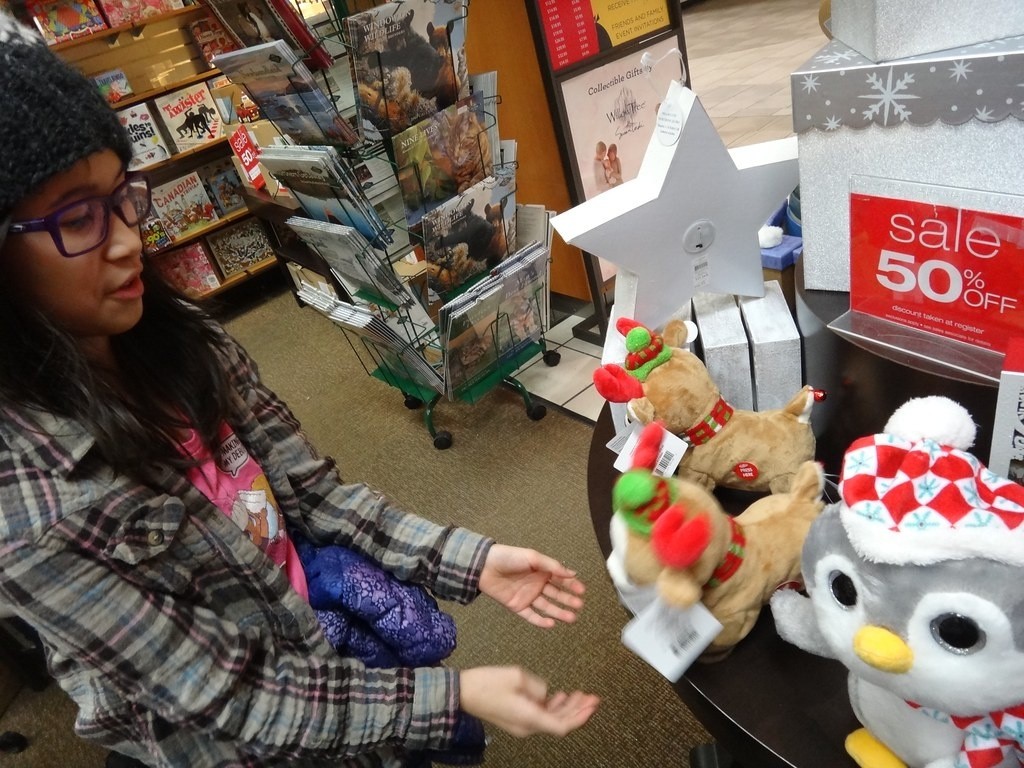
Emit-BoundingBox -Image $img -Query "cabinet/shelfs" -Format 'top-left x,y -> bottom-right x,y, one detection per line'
50,4 -> 276,304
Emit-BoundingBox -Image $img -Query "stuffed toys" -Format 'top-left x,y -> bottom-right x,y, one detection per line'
768,396 -> 1023,768
594,317 -> 817,496
606,462 -> 827,655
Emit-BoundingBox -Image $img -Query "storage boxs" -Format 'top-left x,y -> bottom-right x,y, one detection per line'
689,290 -> 754,417
737,278 -> 802,414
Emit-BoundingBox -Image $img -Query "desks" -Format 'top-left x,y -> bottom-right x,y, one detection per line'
586,250 -> 1004,768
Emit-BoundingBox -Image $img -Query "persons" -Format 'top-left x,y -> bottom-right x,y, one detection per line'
594,141 -> 624,193
0,13 -> 600,768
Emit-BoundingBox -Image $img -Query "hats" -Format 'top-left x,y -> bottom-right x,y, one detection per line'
834,391 -> 1022,569
3,13 -> 131,198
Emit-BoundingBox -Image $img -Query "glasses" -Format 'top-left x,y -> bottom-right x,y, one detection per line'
3,178 -> 156,259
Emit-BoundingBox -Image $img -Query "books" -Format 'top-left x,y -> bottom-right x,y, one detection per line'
0,0 -> 558,401
